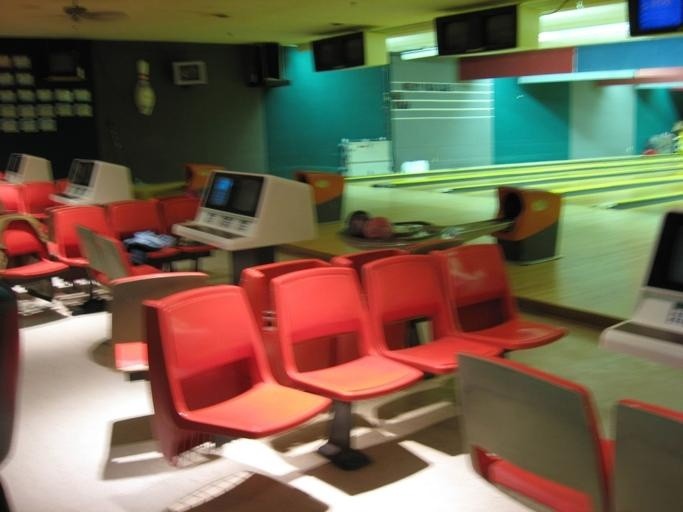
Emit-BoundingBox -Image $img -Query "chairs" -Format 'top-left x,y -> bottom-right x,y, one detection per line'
450,350 -> 683,512
0,181 -> 212,317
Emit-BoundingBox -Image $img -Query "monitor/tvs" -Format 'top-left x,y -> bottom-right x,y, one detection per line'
69,161 -> 94,185
646,212 -> 683,296
435,5 -> 539,55
4,154 -> 21,174
628,0 -> 683,37
204,173 -> 264,218
312,33 -> 388,72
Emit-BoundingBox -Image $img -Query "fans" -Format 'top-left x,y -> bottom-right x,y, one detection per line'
30,0 -> 129,34
